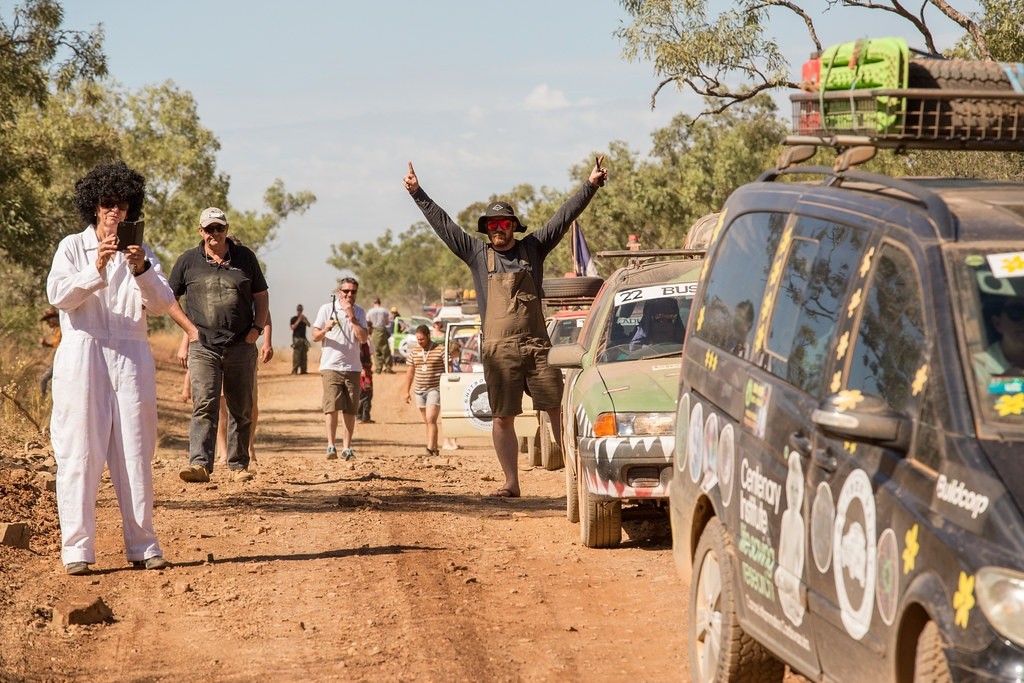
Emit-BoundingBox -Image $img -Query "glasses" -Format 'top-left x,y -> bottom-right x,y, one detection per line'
203,223 -> 226,234
998,302 -> 1024,322
98,195 -> 130,211
340,289 -> 358,295
486,218 -> 513,230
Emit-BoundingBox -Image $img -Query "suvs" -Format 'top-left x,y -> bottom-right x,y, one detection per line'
665,97 -> 1024,683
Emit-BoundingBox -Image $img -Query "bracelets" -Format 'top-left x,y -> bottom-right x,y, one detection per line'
350,316 -> 357,321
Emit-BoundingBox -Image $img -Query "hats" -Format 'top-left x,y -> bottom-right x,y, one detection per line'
474,202 -> 527,233
198,206 -> 228,227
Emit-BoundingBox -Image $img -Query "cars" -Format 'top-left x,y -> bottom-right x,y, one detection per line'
392,298 -> 495,431
544,250 -> 712,548
516,296 -> 599,470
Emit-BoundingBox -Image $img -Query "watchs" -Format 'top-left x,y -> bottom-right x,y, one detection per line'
252,324 -> 264,335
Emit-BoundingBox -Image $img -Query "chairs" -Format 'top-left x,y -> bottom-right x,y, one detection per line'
629,298 -> 685,353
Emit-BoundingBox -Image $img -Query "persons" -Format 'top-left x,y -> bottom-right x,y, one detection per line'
290,304 -> 311,375
365,298 -> 406,374
313,277 -> 369,459
405,324 -> 453,456
177,236 -> 273,464
442,342 -> 464,450
169,207 -> 268,482
647,314 -> 674,344
46,163 -> 169,575
403,155 -> 607,496
986,295 -> 1024,377
429,317 -> 446,344
356,321 -> 375,424
40,310 -> 62,394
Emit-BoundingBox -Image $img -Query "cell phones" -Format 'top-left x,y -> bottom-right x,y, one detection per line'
114,220 -> 144,252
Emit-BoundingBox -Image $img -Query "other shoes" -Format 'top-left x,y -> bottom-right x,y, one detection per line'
341,449 -> 356,461
417,448 -> 437,458
326,447 -> 337,460
357,418 -> 375,424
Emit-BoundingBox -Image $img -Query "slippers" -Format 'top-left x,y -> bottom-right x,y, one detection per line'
486,486 -> 521,500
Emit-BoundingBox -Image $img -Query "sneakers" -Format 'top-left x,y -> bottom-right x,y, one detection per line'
228,467 -> 253,483
64,561 -> 90,575
179,464 -> 210,482
133,556 -> 171,569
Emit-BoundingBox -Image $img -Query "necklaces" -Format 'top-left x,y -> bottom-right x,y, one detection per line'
422,342 -> 434,372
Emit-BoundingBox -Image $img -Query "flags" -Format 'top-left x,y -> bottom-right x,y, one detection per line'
570,222 -> 599,276
